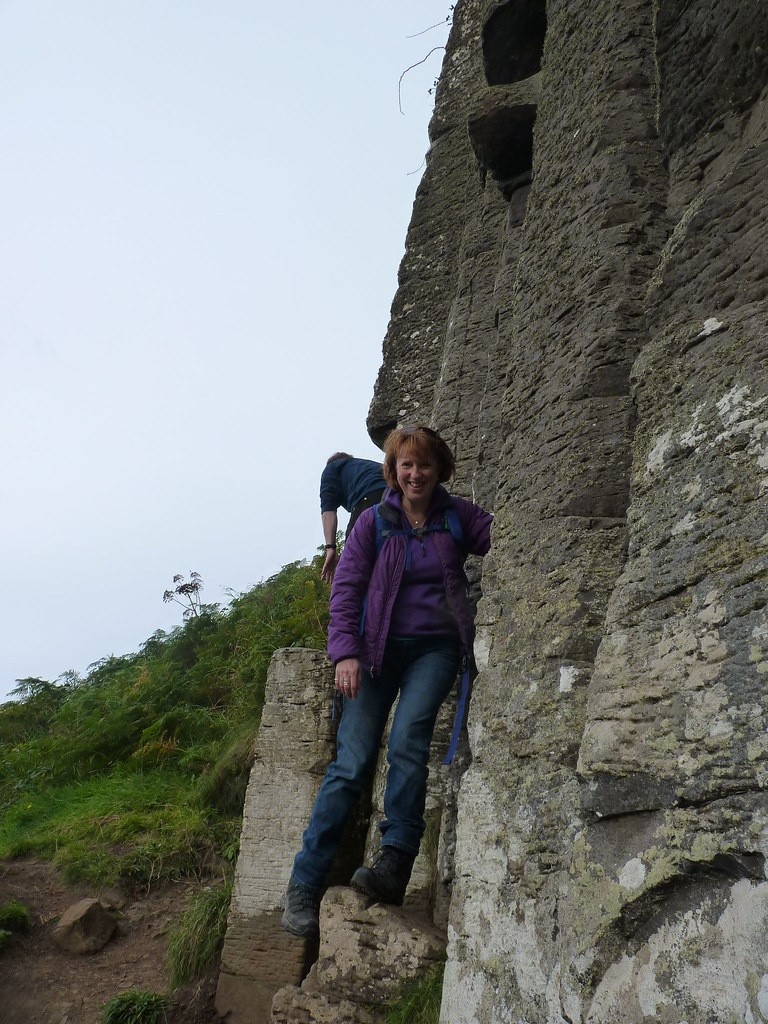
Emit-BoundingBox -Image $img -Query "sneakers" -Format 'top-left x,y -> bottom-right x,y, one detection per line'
279,876 -> 319,938
350,845 -> 415,907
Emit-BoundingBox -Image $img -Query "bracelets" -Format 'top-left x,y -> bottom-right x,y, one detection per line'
324,543 -> 336,550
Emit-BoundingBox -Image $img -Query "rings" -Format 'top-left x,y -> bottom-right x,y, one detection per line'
344,681 -> 351,686
334,678 -> 339,682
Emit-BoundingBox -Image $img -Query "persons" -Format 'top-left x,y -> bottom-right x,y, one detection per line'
280,426 -> 494,941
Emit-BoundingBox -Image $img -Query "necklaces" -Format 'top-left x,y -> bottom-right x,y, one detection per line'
402,506 -> 429,526
